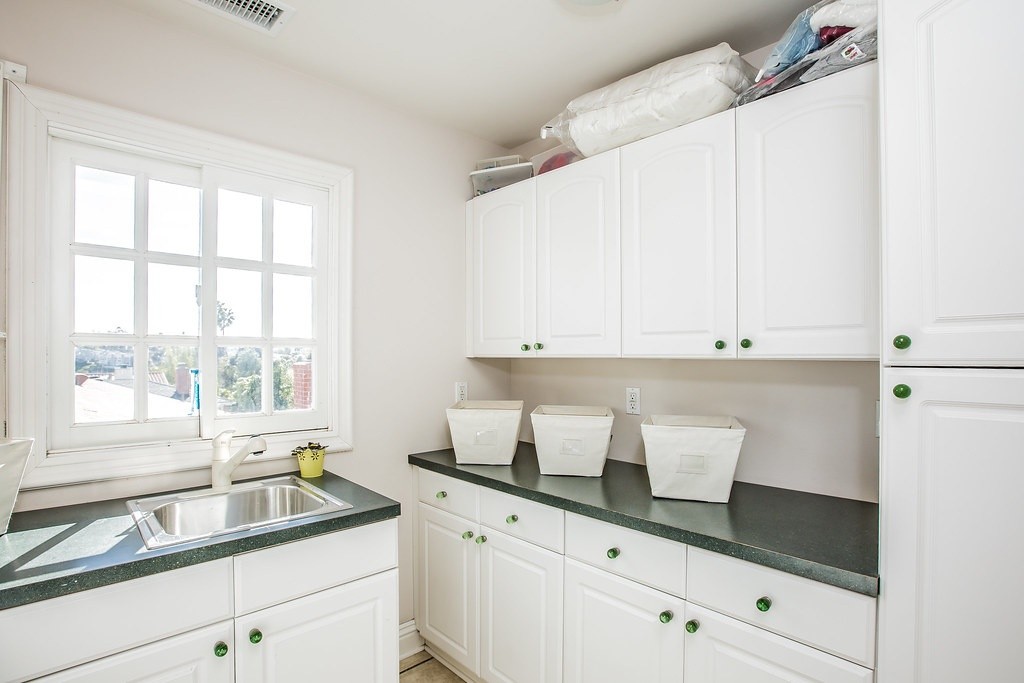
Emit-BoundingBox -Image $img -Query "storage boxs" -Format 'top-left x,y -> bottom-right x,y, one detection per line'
468,139 -> 588,197
0,434 -> 35,536
640,414 -> 747,503
529,404 -> 615,477
446,399 -> 524,467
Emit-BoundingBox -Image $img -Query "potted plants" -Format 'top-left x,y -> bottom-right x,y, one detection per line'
291,442 -> 329,478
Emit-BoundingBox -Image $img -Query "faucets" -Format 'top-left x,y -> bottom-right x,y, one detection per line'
212,430 -> 267,488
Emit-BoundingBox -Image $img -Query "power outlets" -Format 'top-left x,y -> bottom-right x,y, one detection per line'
626,387 -> 641,414
455,381 -> 468,404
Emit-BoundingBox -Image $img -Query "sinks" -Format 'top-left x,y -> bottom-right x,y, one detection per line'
126,474 -> 353,549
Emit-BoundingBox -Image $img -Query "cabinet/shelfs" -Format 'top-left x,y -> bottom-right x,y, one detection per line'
620,57 -> 881,361
411,464 -> 566,683
0,517 -> 400,682
464,146 -> 621,359
877,0 -> 1024,683
562,509 -> 878,683
